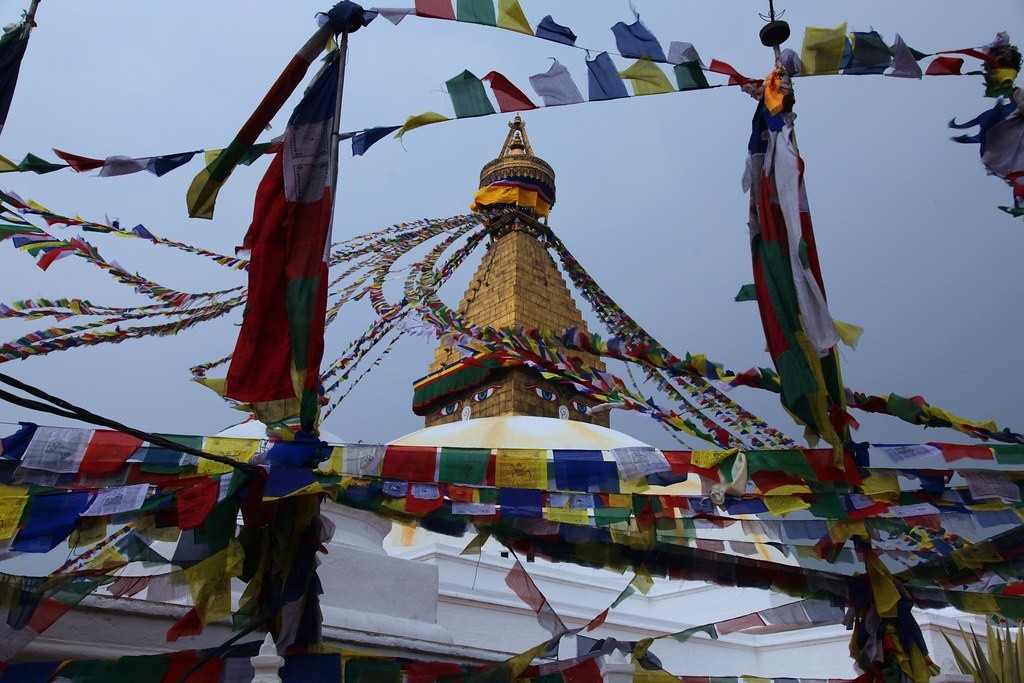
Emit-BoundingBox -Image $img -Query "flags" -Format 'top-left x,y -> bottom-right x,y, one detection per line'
0,0 -> 1024,683
